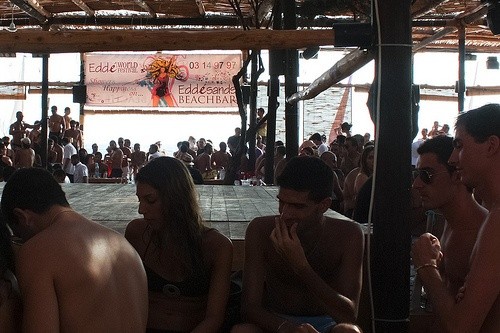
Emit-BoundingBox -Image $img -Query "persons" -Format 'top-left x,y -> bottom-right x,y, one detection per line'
0,166 -> 149,333
0,216 -> 22,333
122,156 -> 233,333
0,106 -> 454,230
227,154 -> 365,333
411,135 -> 491,332
409,103 -> 500,333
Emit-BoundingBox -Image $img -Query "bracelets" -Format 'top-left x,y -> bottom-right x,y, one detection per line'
415,263 -> 440,273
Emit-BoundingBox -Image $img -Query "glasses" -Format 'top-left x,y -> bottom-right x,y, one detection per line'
413,167 -> 449,184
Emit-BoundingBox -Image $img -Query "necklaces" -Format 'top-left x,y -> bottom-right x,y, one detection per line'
50,210 -> 75,224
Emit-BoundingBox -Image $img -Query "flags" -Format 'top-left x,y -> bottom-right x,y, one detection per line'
85,54 -> 244,107
